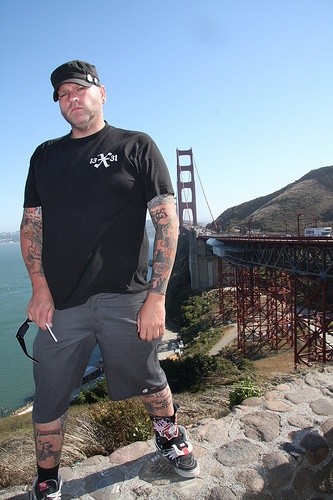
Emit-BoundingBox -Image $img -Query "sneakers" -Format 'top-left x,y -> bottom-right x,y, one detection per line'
30,475 -> 63,500
154,425 -> 200,477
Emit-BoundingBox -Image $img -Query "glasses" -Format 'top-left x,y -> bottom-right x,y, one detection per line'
16,317 -> 58,363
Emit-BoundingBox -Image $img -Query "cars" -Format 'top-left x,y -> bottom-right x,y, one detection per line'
190,225 -> 213,237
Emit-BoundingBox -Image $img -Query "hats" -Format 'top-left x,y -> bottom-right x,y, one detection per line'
51,60 -> 101,102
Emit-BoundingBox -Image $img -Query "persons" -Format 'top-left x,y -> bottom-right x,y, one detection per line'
18,60 -> 199,500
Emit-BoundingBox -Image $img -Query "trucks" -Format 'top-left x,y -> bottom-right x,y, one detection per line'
305,227 -> 332,238
